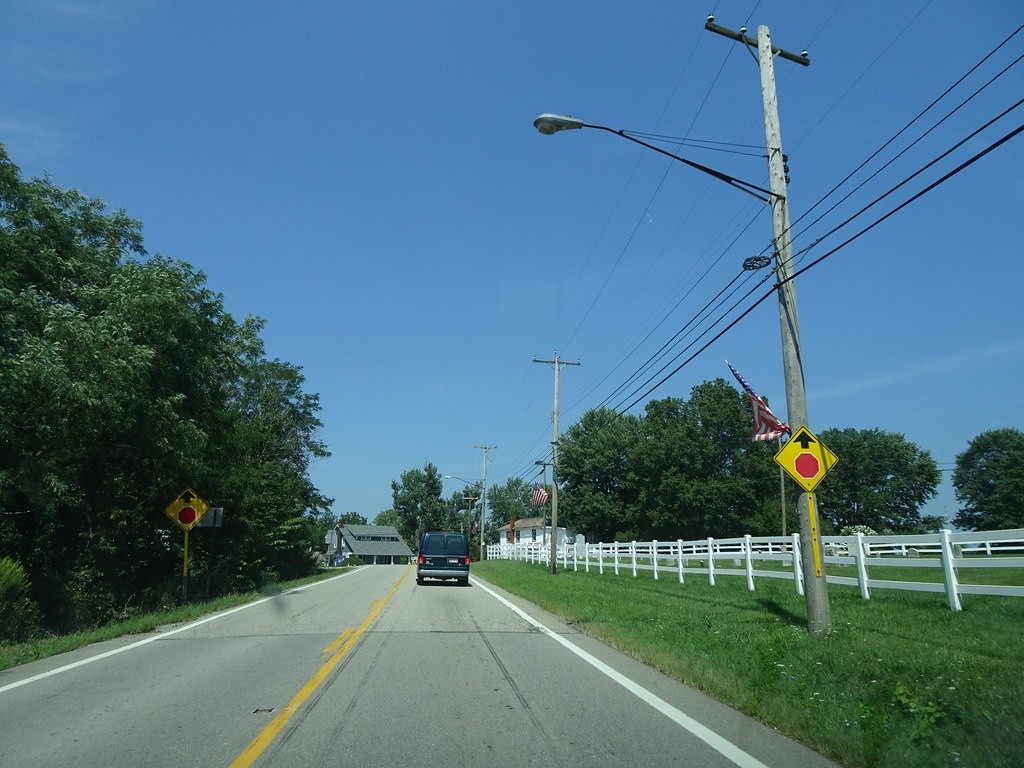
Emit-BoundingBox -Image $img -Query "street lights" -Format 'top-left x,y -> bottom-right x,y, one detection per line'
445,475 -> 486,560
534,114 -> 833,637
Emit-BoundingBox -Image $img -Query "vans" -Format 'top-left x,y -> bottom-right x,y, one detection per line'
416,532 -> 470,586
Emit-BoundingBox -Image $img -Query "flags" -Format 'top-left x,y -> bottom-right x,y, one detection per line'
471,522 -> 479,534
729,364 -> 790,441
531,483 -> 548,506
